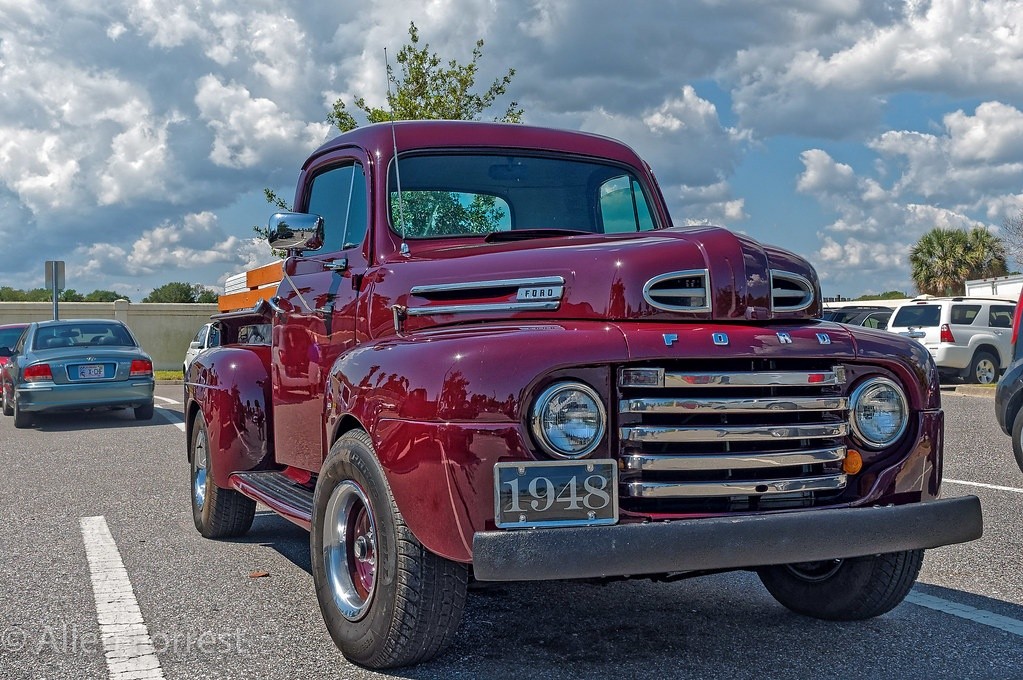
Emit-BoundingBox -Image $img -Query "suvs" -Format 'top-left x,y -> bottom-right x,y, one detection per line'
184,45 -> 984,671
885,293 -> 1017,389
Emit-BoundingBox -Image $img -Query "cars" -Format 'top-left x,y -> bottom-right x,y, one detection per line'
182,322 -> 220,386
265,325 -> 322,400
819,301 -> 902,332
0,317 -> 155,429
0,322 -> 36,407
994,286 -> 1023,475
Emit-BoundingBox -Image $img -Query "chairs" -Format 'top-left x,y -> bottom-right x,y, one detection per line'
996,315 -> 1010,328
38,335 -> 66,347
91,336 -> 123,344
